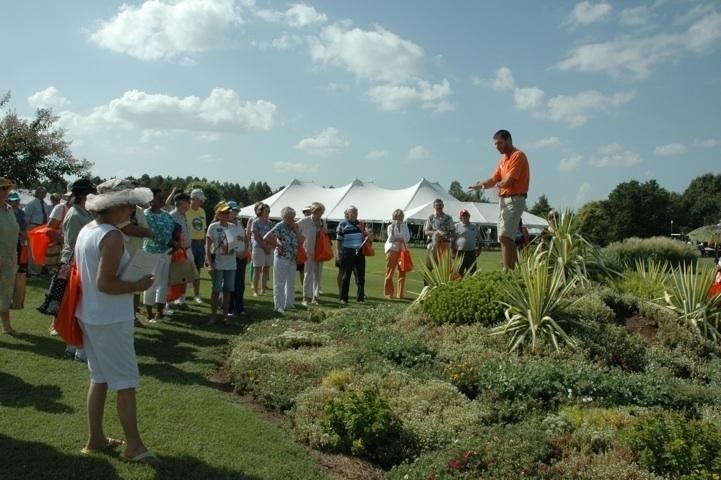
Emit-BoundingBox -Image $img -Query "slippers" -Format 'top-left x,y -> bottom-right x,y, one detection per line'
119,449 -> 169,467
80,436 -> 127,455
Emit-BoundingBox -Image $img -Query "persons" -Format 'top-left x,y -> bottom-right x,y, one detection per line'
514,216 -> 529,265
204,201 -> 333,326
72,178 -> 167,467
539,209 -> 559,253
423,197 -> 457,287
0,176 -> 207,362
382,209 -> 412,300
449,207 -> 482,278
467,128 -> 530,277
336,206 -> 374,304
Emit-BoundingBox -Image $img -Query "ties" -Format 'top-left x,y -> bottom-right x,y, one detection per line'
40,200 -> 47,224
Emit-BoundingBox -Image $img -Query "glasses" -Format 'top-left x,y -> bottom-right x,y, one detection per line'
1,185 -> 11,191
54,199 -> 61,201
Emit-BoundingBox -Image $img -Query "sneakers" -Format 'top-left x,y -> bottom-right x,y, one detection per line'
145,302 -> 194,324
64,348 -> 76,359
192,296 -> 203,304
75,350 -> 88,361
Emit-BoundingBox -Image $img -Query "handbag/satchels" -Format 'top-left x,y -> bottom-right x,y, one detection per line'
314,230 -> 334,262
398,239 -> 415,272
27,224 -> 59,265
169,246 -> 202,286
54,263 -> 84,347
296,241 -> 308,265
11,271 -> 26,310
205,252 -> 216,267
362,239 -> 376,257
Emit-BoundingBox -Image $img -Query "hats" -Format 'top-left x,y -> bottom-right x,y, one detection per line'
213,201 -> 233,215
84,177 -> 155,212
190,187 -> 208,201
173,192 -> 194,201
8,193 -> 20,201
0,176 -> 16,187
460,209 -> 470,218
72,178 -> 97,196
228,200 -> 241,212
62,182 -> 74,198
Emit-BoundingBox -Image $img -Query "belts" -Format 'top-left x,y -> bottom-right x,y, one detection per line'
503,193 -> 527,198
30,222 -> 40,226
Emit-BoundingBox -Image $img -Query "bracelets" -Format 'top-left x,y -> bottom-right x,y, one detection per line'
480,184 -> 485,189
